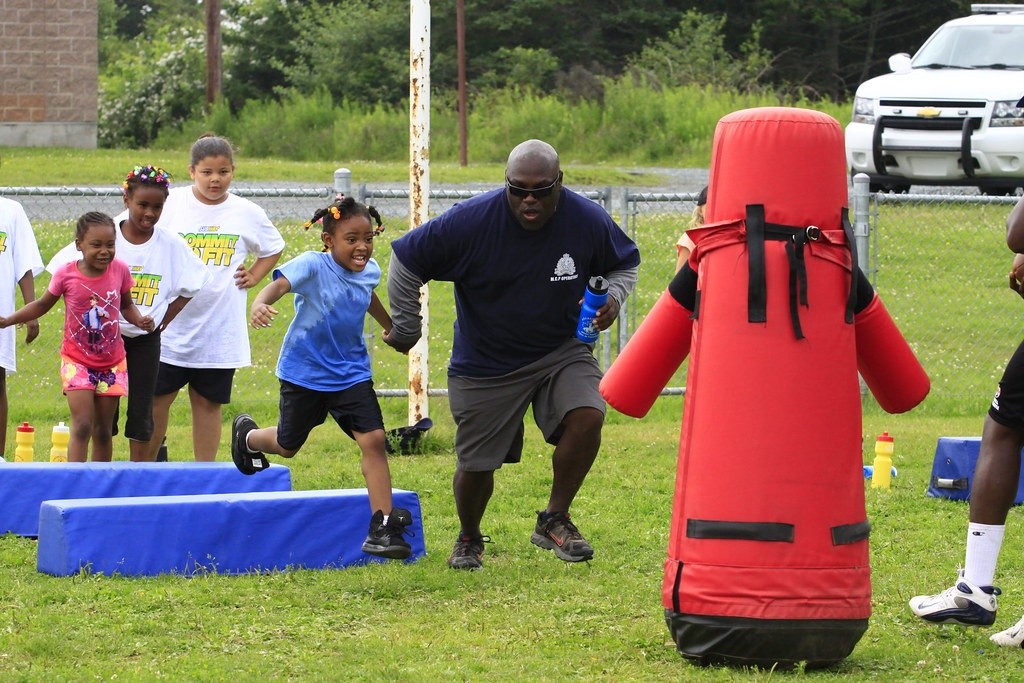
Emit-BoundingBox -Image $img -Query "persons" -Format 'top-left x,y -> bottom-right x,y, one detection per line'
45,164 -> 214,462
382,139 -> 642,572
231,192 -> 415,560
0,212 -> 155,462
909,193 -> 1024,648
112,132 -> 286,462
675,185 -> 708,274
0,197 -> 45,462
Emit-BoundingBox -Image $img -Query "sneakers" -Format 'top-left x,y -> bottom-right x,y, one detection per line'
448,530 -> 495,572
529,508 -> 594,562
909,568 -> 1002,627
361,507 -> 415,559
988,615 -> 1024,649
231,413 -> 271,475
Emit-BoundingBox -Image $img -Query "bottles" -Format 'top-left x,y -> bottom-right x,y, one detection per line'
13,422 -> 35,462
870,432 -> 894,490
863,464 -> 898,480
575,275 -> 610,342
49,420 -> 69,462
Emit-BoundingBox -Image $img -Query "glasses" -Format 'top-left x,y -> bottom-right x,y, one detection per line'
505,171 -> 560,198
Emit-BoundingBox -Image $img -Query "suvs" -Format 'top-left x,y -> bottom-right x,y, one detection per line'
844,3 -> 1023,197
